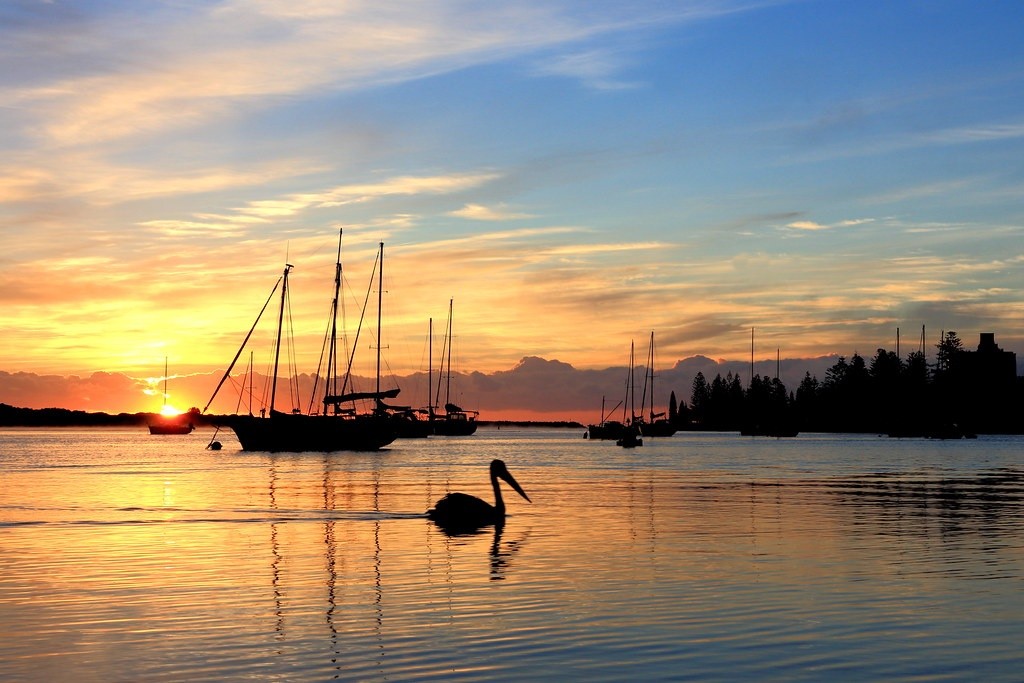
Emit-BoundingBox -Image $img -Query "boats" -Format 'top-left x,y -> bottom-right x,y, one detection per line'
147,420 -> 194,436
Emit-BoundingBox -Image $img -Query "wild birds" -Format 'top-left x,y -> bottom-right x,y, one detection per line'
429,460 -> 533,533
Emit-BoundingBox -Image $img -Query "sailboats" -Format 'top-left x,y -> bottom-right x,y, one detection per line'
230,220 -> 486,453
587,325 -> 675,445
